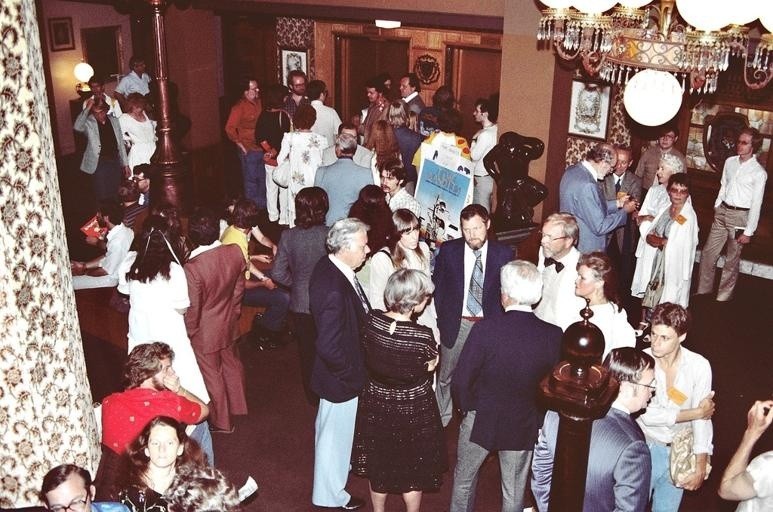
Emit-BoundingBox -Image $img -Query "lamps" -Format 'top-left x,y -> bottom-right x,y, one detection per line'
377,20 -> 401,29
535,0 -> 773,128
75,59 -> 94,96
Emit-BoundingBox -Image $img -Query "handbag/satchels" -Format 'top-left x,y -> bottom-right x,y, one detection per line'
272,158 -> 291,188
670,431 -> 712,488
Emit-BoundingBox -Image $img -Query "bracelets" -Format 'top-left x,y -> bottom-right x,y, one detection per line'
182,388 -> 189,399
260,273 -> 267,282
81,260 -> 87,270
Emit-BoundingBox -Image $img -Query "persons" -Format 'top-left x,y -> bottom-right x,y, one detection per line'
161,463 -> 244,511
530,345 -> 655,511
118,180 -> 147,230
717,398 -> 773,512
642,173 -> 700,344
119,93 -> 158,204
69,196 -> 134,277
269,186 -> 330,408
114,54 -> 150,113
379,157 -> 421,217
383,74 -> 395,97
432,203 -> 516,429
111,415 -> 211,512
597,145 -> 643,256
101,342 -> 215,468
405,109 -> 426,142
362,120 -> 404,187
82,75 -> 122,117
73,96 -> 132,197
633,302 -> 715,512
313,133 -> 375,228
284,70 -> 307,126
395,72 -> 425,114
369,208 -> 441,346
305,80 -> 342,146
255,85 -> 291,226
420,86 -> 464,133
322,121 -> 372,167
696,127 -> 767,303
564,250 -> 637,363
348,184 -> 394,239
277,103 -> 329,230
41,464 -> 131,512
132,163 -> 151,205
220,186 -> 278,264
630,152 -> 693,337
634,126 -> 688,250
307,216 -> 371,510
351,268 -> 450,511
219,198 -> 290,353
387,98 -> 420,195
449,259 -> 564,512
182,210 -> 249,434
559,142 -> 637,254
117,214 -> 211,405
225,74 -> 267,209
358,85 -> 390,151
532,212 -> 586,331
469,98 -> 497,216
412,108 -> 471,180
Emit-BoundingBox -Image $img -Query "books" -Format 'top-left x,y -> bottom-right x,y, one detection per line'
734,226 -> 745,239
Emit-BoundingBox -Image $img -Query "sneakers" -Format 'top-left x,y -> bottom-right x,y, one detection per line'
312,495 -> 365,512
209,423 -> 235,433
251,328 -> 286,348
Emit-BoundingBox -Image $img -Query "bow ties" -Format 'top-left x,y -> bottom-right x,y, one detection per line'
544,257 -> 564,273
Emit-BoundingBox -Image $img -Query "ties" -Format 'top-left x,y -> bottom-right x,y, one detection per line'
466,250 -> 484,318
615,179 -> 620,199
353,276 -> 371,315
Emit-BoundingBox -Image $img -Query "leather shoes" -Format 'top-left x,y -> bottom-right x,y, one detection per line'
693,293 -> 713,303
714,301 -> 734,309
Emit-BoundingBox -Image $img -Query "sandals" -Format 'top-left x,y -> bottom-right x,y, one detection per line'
634,321 -> 650,336
643,331 -> 652,342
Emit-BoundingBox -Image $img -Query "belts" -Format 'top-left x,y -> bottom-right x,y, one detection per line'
721,201 -> 750,211
459,316 -> 481,322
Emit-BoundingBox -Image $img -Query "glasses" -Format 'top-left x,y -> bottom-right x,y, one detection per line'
630,378 -> 657,389
737,141 -> 753,145
538,230 -> 566,244
670,188 -> 688,195
660,135 -> 676,141
51,491 -> 88,512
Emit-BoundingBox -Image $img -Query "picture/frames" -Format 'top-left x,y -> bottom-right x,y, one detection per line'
48,16 -> 75,51
80,24 -> 126,87
279,46 -> 311,89
566,78 -> 610,139
